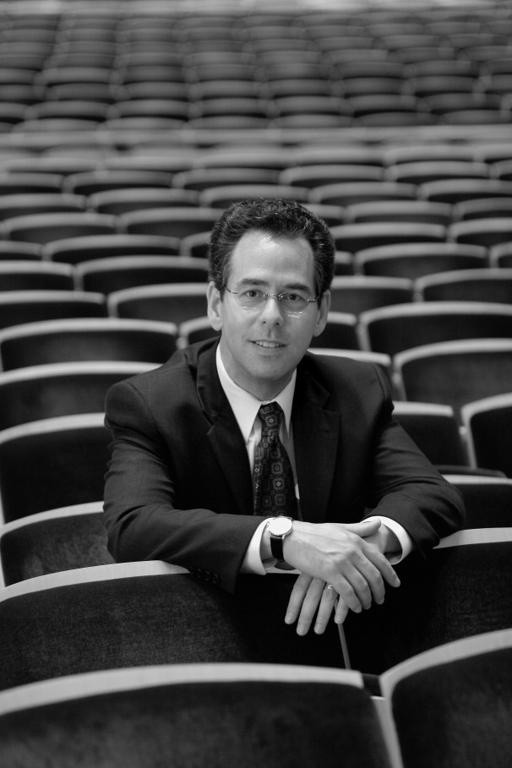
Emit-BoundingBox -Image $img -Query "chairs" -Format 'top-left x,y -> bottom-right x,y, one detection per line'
0,0 -> 512,768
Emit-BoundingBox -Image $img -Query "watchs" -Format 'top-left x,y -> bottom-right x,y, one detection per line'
267,516 -> 293,565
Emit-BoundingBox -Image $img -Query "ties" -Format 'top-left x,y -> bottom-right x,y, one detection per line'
252,403 -> 300,521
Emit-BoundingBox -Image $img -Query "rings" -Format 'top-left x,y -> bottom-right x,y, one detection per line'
324,583 -> 335,589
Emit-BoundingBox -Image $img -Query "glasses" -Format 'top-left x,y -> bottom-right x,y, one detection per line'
224,285 -> 320,309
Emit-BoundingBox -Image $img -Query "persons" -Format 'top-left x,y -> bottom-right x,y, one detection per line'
103,197 -> 465,635
